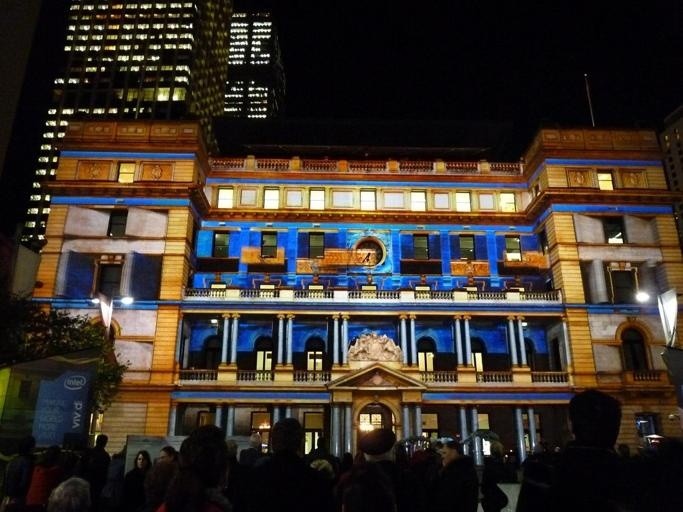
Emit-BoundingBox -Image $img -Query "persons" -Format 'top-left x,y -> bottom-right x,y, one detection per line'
415,275 -> 430,286
361,275 -> 378,286
308,273 -> 323,285
212,273 -> 225,284
260,273 -> 275,285
511,275 -> 524,287
463,275 -> 478,287
